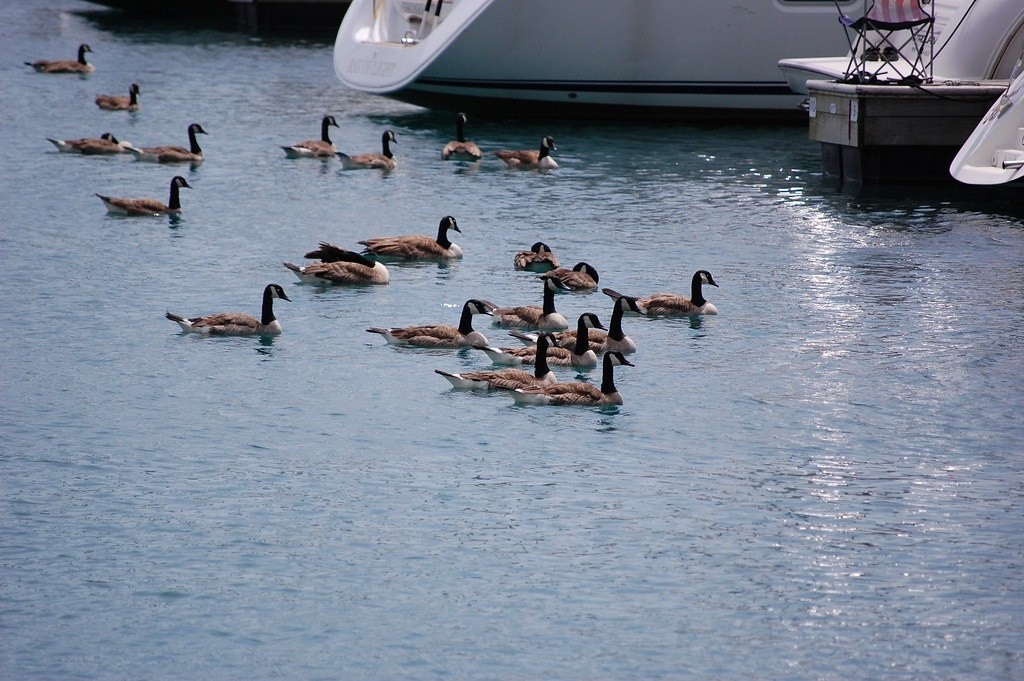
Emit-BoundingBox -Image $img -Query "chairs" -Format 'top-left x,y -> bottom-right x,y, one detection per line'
834,0 -> 936,86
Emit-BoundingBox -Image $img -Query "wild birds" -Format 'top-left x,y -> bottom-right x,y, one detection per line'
365,278 -> 645,406
280,115 -> 340,158
493,136 -> 559,169
122,124 -> 208,163
96,83 -> 140,112
356,216 -> 464,259
165,283 -> 292,335
46,133 -> 131,154
24,44 -> 95,73
514,242 -> 599,293
334,130 -> 398,170
601,269 -> 720,317
95,175 -> 193,217
282,240 -> 389,285
441,112 -> 482,161
